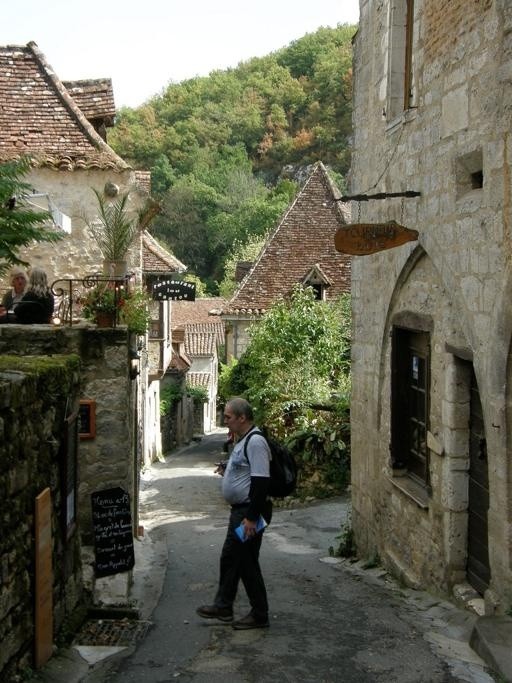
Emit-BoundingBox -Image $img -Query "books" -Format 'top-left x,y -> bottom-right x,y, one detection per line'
234,514 -> 268,543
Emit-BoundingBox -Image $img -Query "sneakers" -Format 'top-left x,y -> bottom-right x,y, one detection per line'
194,603 -> 236,623
231,605 -> 272,630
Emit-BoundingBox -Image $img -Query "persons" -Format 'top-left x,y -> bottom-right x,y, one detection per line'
221,427 -> 234,454
0,270 -> 31,321
14,269 -> 54,323
197,398 -> 273,630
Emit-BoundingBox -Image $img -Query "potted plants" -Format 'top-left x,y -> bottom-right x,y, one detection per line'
84,184 -> 161,327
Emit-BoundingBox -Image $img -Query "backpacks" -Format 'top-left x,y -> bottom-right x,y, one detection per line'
243,429 -> 299,499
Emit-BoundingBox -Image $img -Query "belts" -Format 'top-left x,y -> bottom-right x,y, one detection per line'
231,501 -> 254,509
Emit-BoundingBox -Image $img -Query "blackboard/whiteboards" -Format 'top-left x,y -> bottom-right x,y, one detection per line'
91,487 -> 134,578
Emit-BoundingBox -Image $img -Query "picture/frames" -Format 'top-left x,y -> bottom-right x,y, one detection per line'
78,398 -> 95,440
